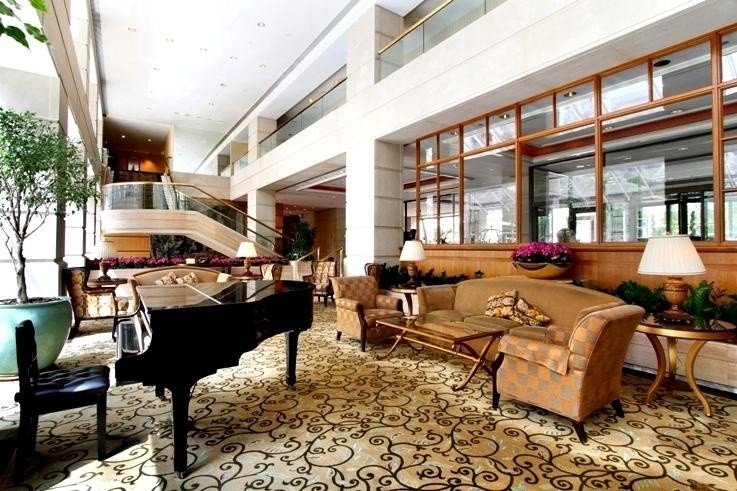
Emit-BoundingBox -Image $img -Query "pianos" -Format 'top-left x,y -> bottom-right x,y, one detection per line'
111,281 -> 316,477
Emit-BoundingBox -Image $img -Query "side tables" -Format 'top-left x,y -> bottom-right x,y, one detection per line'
634,316 -> 737,418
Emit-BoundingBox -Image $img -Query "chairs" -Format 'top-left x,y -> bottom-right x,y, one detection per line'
63,267 -> 128,342
492,304 -> 645,444
14,320 -> 110,481
329,275 -> 406,352
131,261 -> 336,307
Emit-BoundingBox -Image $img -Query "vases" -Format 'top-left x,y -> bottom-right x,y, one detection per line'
512,262 -> 573,279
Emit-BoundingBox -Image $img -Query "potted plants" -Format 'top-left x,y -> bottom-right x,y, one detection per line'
288,221 -> 318,261
0,106 -> 106,382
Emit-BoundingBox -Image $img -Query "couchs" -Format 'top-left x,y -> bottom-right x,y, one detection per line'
416,275 -> 625,361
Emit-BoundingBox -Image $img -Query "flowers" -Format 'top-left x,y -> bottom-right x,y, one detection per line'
510,240 -> 572,261
89,255 -> 288,270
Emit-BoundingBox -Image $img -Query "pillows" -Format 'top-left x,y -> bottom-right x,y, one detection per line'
156,272 -> 200,284
484,289 -> 551,326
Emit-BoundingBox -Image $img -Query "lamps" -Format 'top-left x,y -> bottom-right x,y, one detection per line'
89,241 -> 120,283
400,240 -> 426,286
236,242 -> 258,275
637,235 -> 707,323
